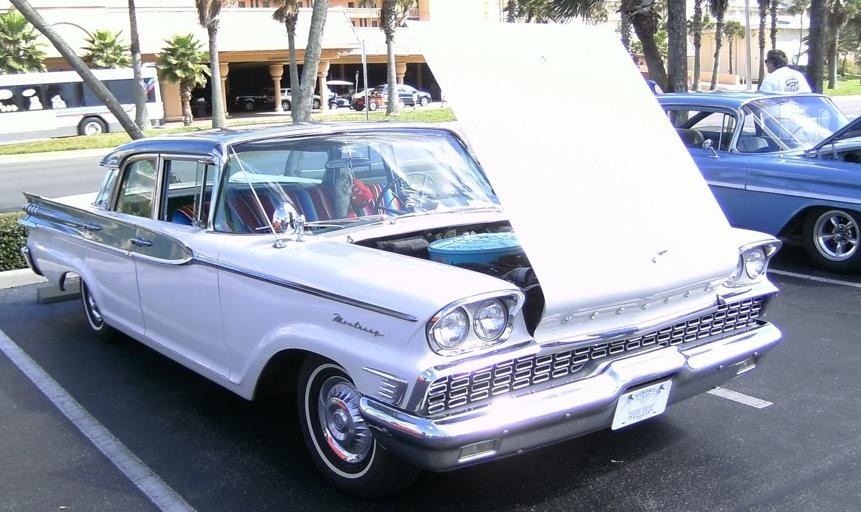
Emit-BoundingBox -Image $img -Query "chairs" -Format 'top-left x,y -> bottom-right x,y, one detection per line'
736,117 -> 804,153
225,177 -> 399,234
170,198 -> 210,226
677,127 -> 705,148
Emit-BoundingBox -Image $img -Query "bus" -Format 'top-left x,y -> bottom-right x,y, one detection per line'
0,61 -> 164,145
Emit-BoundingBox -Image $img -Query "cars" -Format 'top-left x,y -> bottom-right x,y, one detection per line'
645,80 -> 861,272
235,86 -> 275,113
328,82 -> 432,112
16,22 -> 783,495
280,87 -> 320,111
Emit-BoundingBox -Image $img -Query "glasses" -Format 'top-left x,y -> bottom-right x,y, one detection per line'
765,60 -> 767,63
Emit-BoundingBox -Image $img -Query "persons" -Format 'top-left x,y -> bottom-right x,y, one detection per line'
759,49 -> 814,97
